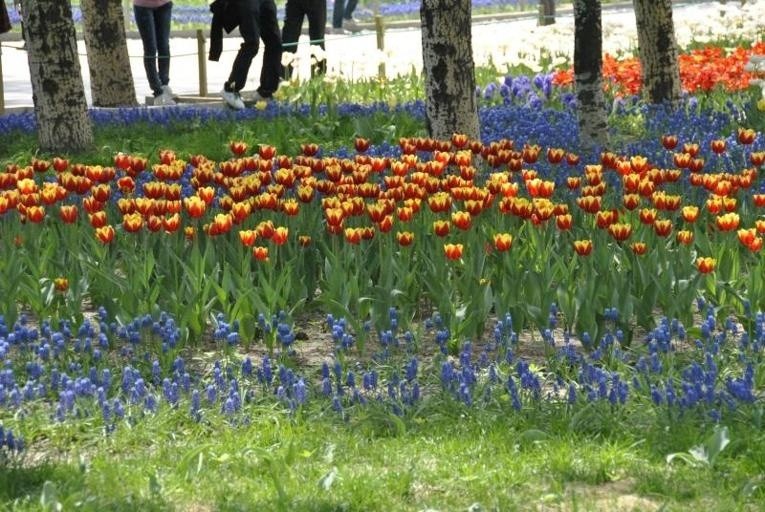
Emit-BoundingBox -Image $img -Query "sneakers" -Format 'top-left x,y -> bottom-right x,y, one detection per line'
253,93 -> 267,102
153,85 -> 176,105
220,89 -> 244,109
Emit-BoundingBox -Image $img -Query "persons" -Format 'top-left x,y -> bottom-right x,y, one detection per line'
281,1 -> 328,80
220,1 -> 283,109
332,1 -> 362,34
0,1 -> 12,113
133,0 -> 179,107
16,0 -> 28,50
